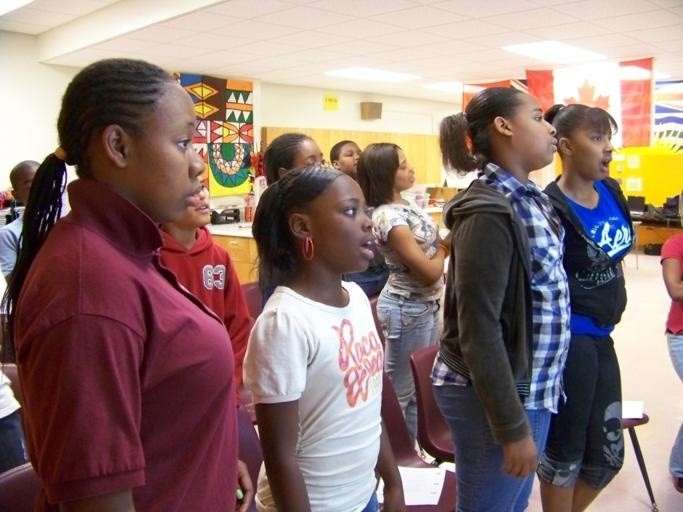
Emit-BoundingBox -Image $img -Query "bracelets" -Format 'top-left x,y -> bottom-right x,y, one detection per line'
436,243 -> 448,259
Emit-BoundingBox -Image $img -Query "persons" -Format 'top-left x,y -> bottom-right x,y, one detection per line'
536,104 -> 632,512
330,141 -> 389,297
430,86 -> 571,512
660,234 -> 683,493
261,133 -> 327,309
0,268 -> 27,474
154,175 -> 263,512
242,165 -> 407,512
0,59 -> 256,512
0,160 -> 66,285
357,143 -> 452,448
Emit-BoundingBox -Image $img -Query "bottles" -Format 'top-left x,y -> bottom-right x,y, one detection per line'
414,193 -> 431,209
249,185 -> 255,196
5,205 -> 20,225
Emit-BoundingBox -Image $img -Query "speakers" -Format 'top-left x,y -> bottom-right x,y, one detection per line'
360,102 -> 382,119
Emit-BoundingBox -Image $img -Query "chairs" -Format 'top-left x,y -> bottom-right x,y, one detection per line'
407,346 -> 460,468
378,370 -> 462,511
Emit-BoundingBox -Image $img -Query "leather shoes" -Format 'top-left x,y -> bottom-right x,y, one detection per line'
674,476 -> 683,492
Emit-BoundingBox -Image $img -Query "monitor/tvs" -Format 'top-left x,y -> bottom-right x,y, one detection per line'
628,196 -> 645,212
